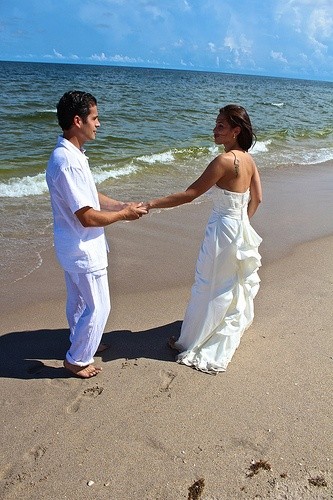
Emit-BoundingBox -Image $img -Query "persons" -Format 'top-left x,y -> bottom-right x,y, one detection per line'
138,105 -> 263,376
46,90 -> 148,378
